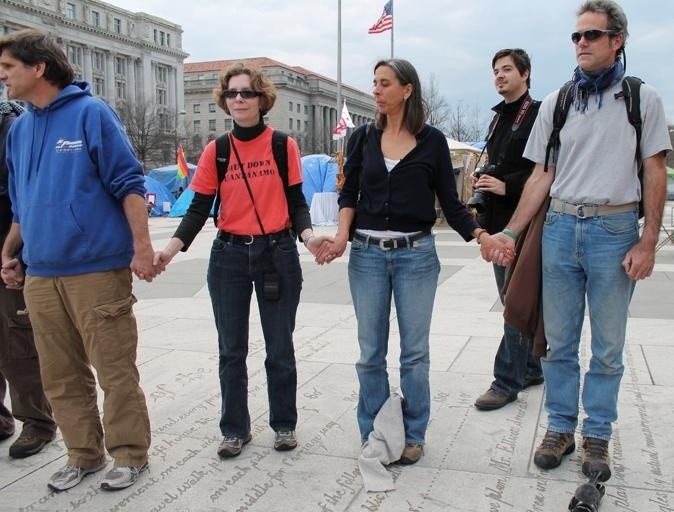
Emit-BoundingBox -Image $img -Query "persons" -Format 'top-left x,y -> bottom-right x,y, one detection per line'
0,29 -> 166,491
1,100 -> 57,458
489,1 -> 673,482
315,59 -> 515,464
139,63 -> 337,456
468,49 -> 544,409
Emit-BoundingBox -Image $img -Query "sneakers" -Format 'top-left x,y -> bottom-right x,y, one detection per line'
273,427 -> 298,450
473,371 -> 545,409
46,456 -> 108,490
399,443 -> 423,465
533,428 -> 575,471
100,456 -> 150,489
581,435 -> 612,482
9,423 -> 58,458
216,431 -> 252,456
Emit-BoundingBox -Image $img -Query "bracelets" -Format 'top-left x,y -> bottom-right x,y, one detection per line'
304,234 -> 313,246
477,229 -> 486,244
502,228 -> 519,241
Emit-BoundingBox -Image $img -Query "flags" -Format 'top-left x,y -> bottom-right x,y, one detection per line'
332,97 -> 356,141
368,0 -> 392,34
175,144 -> 189,180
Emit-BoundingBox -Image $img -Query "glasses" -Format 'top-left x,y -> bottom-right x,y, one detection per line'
224,89 -> 260,99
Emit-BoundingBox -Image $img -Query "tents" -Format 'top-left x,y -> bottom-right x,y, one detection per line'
132,163 -> 218,218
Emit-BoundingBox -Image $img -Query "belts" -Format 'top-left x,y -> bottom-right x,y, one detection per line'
547,195 -> 640,218
215,227 -> 291,246
352,229 -> 429,249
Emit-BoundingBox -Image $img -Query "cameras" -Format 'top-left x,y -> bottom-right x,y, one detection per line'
467,163 -> 497,214
